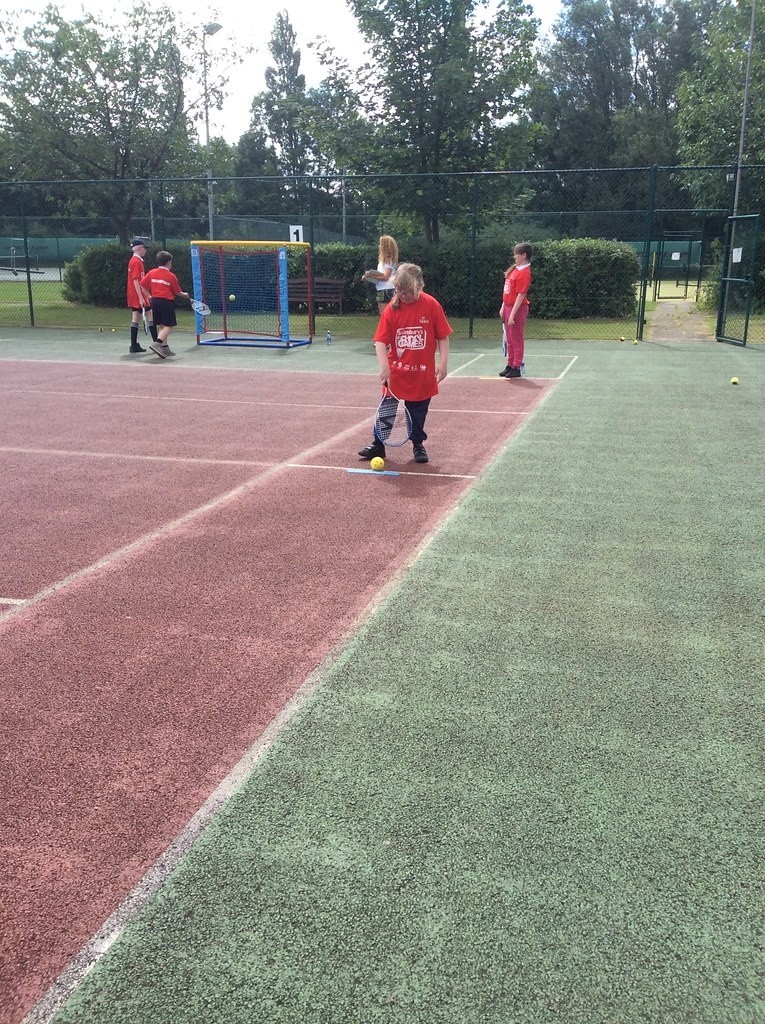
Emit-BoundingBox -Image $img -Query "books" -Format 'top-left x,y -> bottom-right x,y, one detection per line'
362,269 -> 383,285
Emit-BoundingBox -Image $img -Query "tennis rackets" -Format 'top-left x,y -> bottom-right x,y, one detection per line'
187,295 -> 212,316
373,380 -> 413,448
141,305 -> 150,336
501,320 -> 508,357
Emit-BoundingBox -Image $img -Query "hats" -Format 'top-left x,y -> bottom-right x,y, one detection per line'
130,238 -> 150,248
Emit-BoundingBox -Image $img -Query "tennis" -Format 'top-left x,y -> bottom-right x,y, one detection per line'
619,336 -> 625,342
98,326 -> 105,332
730,376 -> 740,386
111,328 -> 116,333
229,294 -> 236,302
633,339 -> 639,345
370,456 -> 385,471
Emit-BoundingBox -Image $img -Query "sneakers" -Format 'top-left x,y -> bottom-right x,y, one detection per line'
357,441 -> 386,458
148,341 -> 167,359
412,443 -> 428,462
161,345 -> 176,356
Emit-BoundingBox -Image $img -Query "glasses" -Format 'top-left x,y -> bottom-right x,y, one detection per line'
394,288 -> 415,297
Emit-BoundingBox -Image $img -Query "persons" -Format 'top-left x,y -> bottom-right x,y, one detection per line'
499,243 -> 534,379
358,263 -> 454,464
127,235 -> 158,353
366,235 -> 399,315
138,251 -> 189,357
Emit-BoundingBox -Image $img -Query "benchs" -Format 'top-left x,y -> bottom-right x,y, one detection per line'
287,277 -> 345,315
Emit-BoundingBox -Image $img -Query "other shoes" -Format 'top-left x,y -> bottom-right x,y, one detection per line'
505,367 -> 522,378
499,365 -> 512,376
129,342 -> 146,353
